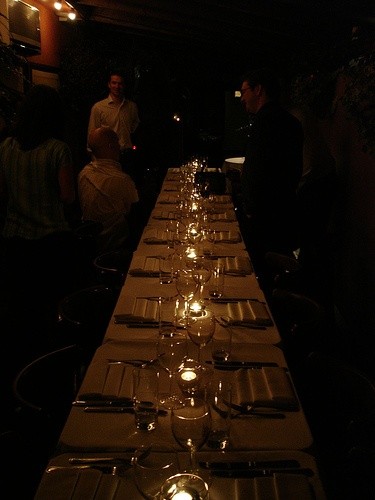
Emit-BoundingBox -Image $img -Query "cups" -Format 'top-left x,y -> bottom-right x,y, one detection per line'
205,380 -> 233,450
159,472 -> 210,500
159,254 -> 173,285
210,315 -> 233,364
209,263 -> 225,298
135,367 -> 158,431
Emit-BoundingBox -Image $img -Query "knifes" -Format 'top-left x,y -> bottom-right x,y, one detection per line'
200,238 -> 314,478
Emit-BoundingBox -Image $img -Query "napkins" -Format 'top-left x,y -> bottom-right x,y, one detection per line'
36,193 -> 314,500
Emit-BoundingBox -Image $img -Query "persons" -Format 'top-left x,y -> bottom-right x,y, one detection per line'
0,72 -> 143,413
240,70 -> 340,358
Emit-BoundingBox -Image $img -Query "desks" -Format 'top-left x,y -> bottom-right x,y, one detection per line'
36,449 -> 325,500
102,286 -> 282,345
122,251 -> 260,288
135,225 -> 246,250
146,167 -> 237,228
56,339 -> 314,450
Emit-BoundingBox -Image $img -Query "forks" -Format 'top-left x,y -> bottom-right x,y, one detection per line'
47,217 -> 173,476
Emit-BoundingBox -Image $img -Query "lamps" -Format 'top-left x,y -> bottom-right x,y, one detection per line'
56,11 -> 77,21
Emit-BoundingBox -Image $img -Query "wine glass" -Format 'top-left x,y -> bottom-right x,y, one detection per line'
187,310 -> 213,373
175,268 -> 200,326
192,256 -> 213,308
170,397 -> 213,488
158,331 -> 188,407
132,445 -> 179,500
177,156 -> 214,286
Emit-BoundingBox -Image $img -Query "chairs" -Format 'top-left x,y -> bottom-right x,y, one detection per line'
93,251 -> 133,290
255,251 -> 298,298
267,288 -> 321,359
12,344 -> 82,456
57,285 -> 121,347
